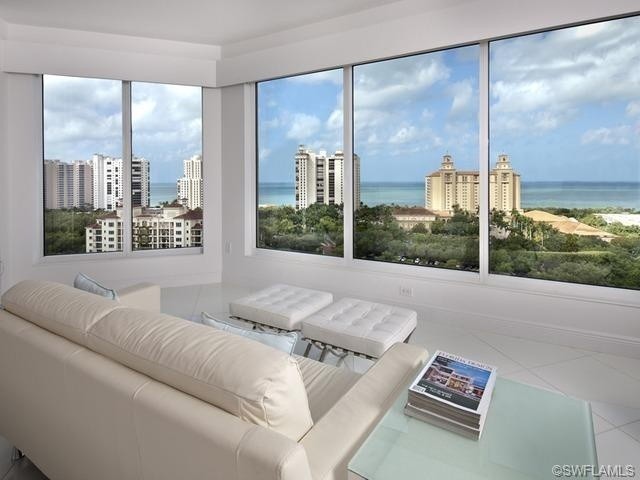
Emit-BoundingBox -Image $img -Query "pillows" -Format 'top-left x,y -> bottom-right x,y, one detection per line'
71,270 -> 298,364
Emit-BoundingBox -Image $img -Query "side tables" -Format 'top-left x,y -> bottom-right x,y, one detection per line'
341,370 -> 601,480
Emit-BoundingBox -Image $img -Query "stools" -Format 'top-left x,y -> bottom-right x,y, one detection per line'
227,281 -> 419,370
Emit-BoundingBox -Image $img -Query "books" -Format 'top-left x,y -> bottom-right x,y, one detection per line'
403,347 -> 499,443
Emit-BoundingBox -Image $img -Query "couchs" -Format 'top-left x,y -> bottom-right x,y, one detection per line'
0,279 -> 427,480
